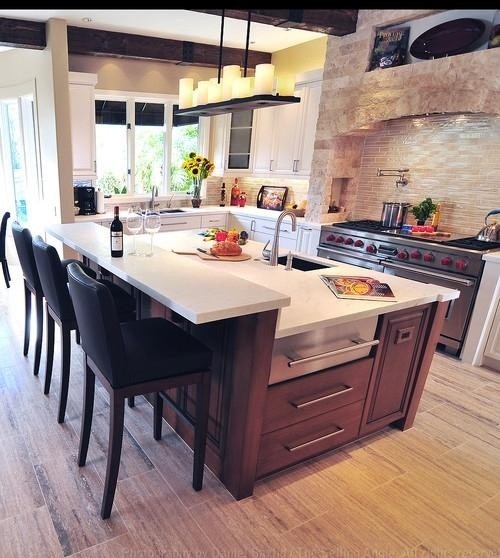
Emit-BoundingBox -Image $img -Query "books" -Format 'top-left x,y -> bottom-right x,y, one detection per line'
320,273 -> 397,302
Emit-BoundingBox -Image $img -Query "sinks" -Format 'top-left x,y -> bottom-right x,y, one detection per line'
263,253 -> 330,272
164,209 -> 184,213
142,210 -> 165,216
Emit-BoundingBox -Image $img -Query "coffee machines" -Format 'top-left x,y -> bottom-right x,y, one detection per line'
74,186 -> 96,215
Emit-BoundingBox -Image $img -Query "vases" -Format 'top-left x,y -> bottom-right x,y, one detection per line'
191,178 -> 202,208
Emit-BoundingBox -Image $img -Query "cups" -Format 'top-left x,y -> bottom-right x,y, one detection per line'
238,237 -> 245,245
215,232 -> 226,241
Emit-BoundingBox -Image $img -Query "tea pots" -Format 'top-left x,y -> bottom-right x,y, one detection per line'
477,209 -> 500,245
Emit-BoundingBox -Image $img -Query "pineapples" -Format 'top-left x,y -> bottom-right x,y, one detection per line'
228,231 -> 239,242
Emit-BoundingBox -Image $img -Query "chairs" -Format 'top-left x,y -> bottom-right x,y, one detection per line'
12,221 -> 44,375
35,234 -> 137,427
63,261 -> 213,520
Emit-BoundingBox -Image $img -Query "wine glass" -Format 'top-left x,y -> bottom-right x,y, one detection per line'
144,207 -> 161,258
126,206 -> 143,257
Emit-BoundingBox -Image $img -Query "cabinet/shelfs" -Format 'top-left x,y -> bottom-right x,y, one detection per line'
146,213 -> 202,230
227,210 -> 251,241
200,210 -> 229,232
253,75 -> 289,175
275,67 -> 324,175
359,292 -> 450,447
250,308 -> 386,483
251,215 -> 298,253
67,70 -> 101,179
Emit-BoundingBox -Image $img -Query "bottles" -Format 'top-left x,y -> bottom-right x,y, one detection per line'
239,192 -> 246,207
220,182 -> 226,206
230,177 -> 240,206
111,206 -> 123,256
96,188 -> 106,215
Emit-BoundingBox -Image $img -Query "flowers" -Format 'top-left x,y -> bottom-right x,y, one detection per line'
181,149 -> 215,180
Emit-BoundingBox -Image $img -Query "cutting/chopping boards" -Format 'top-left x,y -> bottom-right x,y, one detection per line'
172,248 -> 251,261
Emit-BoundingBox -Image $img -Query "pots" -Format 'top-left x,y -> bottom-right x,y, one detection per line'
381,202 -> 412,227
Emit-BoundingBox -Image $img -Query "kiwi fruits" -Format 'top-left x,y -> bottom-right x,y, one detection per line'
238,239 -> 246,245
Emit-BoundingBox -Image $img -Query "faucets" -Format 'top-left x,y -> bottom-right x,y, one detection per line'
150,185 -> 160,210
269,209 -> 296,266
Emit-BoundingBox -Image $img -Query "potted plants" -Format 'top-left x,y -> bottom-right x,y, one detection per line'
410,197 -> 436,226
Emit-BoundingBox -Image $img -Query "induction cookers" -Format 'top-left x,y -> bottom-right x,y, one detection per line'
332,219 -> 400,232
442,236 -> 500,251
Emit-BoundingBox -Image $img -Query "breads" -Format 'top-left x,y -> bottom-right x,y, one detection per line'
210,240 -> 242,256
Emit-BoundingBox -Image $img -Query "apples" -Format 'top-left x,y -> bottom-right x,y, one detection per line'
214,231 -> 228,242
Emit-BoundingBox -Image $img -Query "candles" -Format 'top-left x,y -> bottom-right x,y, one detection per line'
178,64 -> 295,109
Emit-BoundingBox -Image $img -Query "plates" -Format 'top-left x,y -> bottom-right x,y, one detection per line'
410,17 -> 485,60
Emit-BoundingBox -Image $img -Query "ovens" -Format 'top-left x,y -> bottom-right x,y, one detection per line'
316,225 -> 480,356
